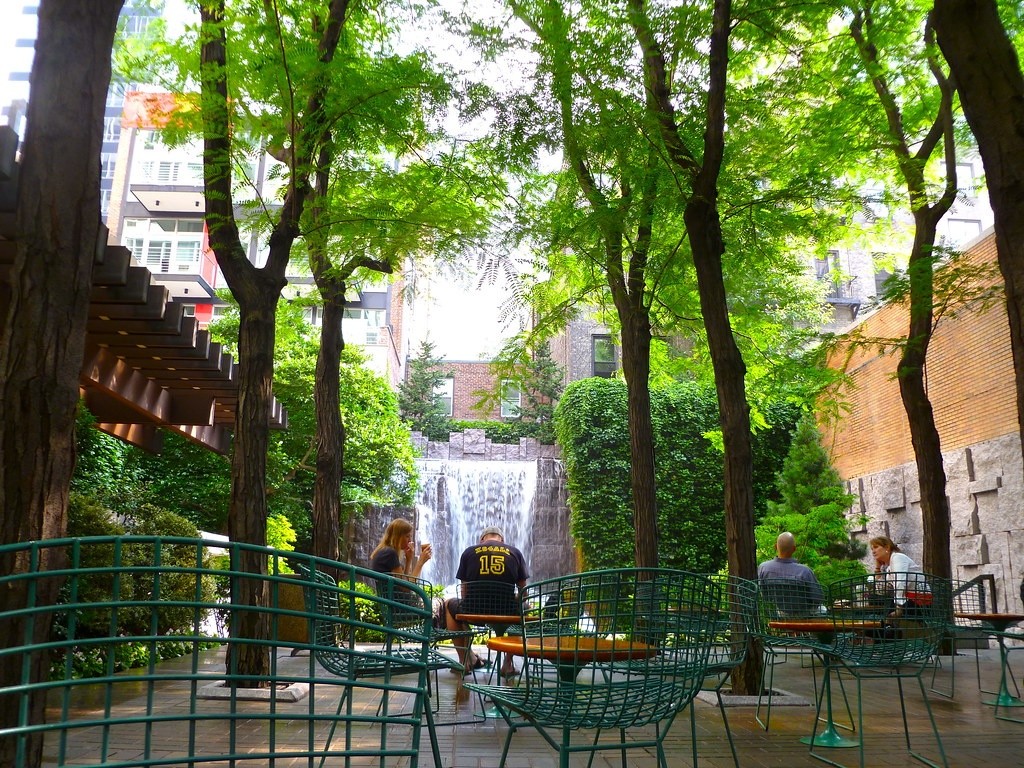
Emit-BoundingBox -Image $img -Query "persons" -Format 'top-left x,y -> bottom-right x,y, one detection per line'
757,531 -> 840,663
446,526 -> 530,678
849,536 -> 932,646
370,519 -> 494,669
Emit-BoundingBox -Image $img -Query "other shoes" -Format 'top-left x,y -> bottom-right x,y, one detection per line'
475,652 -> 493,664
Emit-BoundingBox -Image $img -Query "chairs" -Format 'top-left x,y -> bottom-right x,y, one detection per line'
0,534 -> 444,768
297,563 -> 487,728
374,568 -> 1024,768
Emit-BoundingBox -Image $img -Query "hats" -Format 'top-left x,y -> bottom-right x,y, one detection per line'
481,526 -> 504,540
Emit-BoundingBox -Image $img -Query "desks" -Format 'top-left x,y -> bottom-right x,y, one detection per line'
768,620 -> 881,748
488,634 -> 656,768
455,614 -> 540,719
953,613 -> 1024,708
829,605 -> 886,676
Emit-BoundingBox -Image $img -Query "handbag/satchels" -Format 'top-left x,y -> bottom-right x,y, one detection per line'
875,625 -> 903,644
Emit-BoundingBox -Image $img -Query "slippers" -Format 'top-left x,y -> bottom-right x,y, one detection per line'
465,657 -> 485,669
500,668 -> 521,676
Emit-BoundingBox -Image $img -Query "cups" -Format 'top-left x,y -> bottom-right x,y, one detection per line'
421,544 -> 431,559
